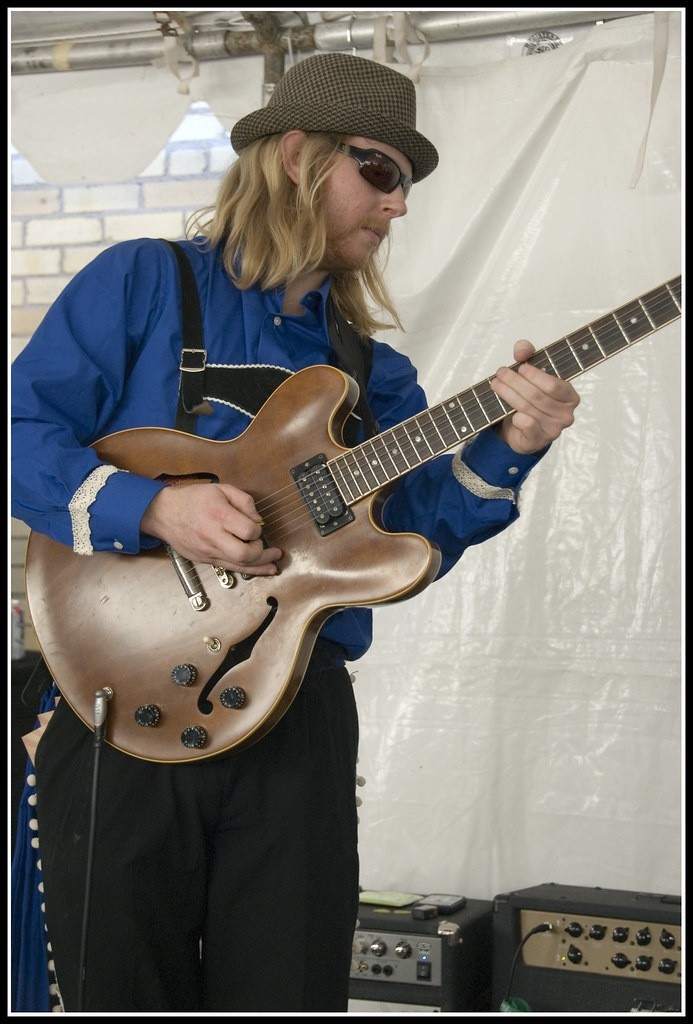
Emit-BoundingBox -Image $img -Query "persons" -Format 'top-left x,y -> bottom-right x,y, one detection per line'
11,54 -> 579,1012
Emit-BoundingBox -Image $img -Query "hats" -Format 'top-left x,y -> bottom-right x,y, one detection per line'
230,53 -> 439,184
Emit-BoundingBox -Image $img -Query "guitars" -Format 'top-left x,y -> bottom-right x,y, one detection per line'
27,274 -> 682,768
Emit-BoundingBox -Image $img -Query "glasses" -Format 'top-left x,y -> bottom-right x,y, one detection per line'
328,139 -> 413,203
